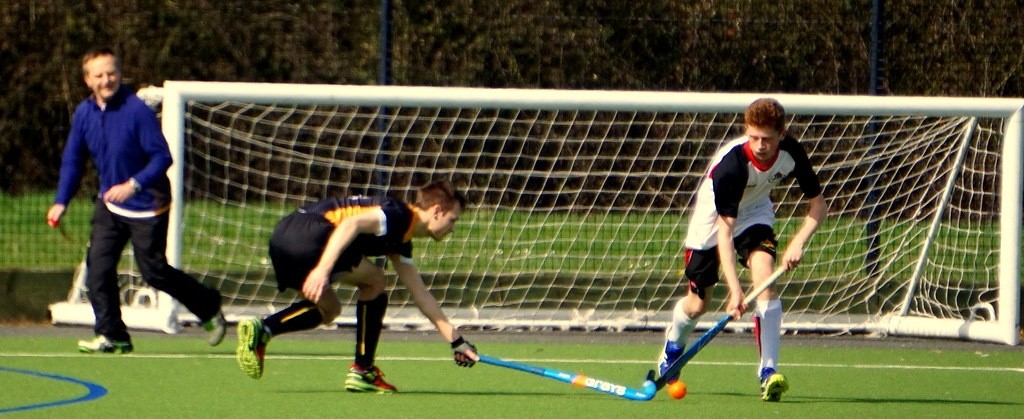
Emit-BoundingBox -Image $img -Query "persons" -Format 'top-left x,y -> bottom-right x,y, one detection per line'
658,98 -> 828,402
237,180 -> 480,394
46,47 -> 228,355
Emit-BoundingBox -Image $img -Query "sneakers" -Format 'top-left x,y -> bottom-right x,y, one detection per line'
237,317 -> 271,380
344,360 -> 398,395
77,332 -> 133,353
657,322 -> 687,385
760,365 -> 788,400
203,312 -> 226,346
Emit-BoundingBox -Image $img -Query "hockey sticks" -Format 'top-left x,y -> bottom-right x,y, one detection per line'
646,265 -> 787,391
478,355 -> 656,401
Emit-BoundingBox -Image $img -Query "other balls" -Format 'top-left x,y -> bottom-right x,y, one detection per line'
668,382 -> 686,399
